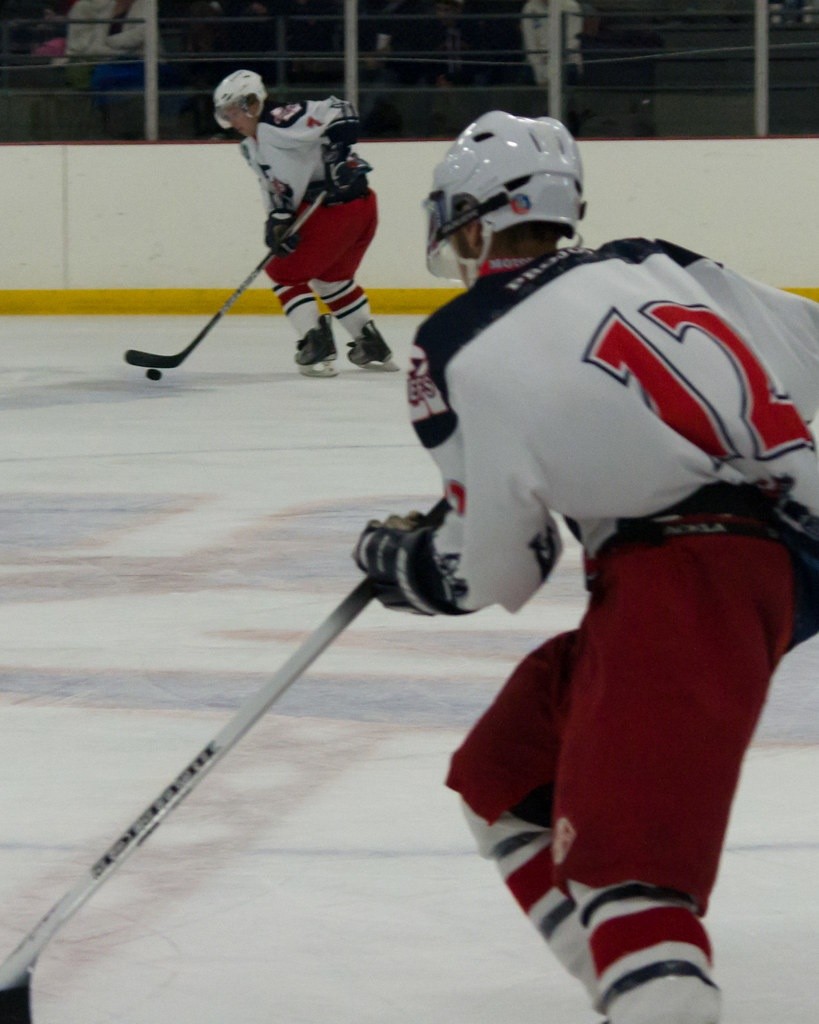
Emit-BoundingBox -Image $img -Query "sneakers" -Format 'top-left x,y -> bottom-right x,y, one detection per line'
345,331 -> 401,375
293,328 -> 342,380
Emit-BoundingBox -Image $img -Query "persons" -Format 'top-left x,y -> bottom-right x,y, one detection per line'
352,111 -> 819,1024
217,2 -> 287,82
213,70 -> 400,378
521,0 -> 588,138
64,0 -> 168,139
418,1 -> 491,139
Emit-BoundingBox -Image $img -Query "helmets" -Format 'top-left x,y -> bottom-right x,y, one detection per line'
211,67 -> 270,109
427,106 -> 589,226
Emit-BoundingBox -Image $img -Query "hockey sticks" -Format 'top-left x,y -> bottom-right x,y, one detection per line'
1,497 -> 457,1023
123,153 -> 359,368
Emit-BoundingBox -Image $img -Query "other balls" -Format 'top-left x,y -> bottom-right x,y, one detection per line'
146,369 -> 162,381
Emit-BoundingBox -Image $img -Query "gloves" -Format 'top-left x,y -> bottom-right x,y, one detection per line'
352,508 -> 454,624
321,145 -> 358,195
263,209 -> 301,262
